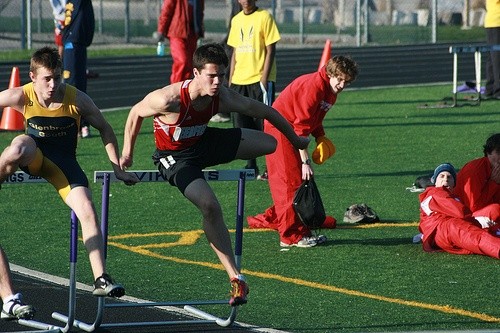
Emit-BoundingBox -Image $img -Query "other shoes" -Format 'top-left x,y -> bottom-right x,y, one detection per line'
257,171 -> 268,180
240,164 -> 259,176
78,126 -> 90,138
210,112 -> 231,122
482,91 -> 500,100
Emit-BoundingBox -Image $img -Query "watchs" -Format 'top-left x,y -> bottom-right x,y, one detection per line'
301,158 -> 311,165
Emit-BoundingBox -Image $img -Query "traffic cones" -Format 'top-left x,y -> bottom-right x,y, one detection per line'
0,66 -> 25,130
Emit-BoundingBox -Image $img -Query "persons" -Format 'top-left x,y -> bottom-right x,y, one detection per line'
418,133 -> 500,260
483,0 -> 500,100
0,245 -> 35,319
119,44 -> 310,304
61,0 -> 95,137
152,0 -> 206,85
246,55 -> 358,247
227,0 -> 281,133
0,46 -> 137,297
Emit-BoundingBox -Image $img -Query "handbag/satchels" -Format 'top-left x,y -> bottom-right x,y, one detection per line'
292,174 -> 326,226
415,170 -> 435,188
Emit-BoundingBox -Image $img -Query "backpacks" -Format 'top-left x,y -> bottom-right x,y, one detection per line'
71,0 -> 95,46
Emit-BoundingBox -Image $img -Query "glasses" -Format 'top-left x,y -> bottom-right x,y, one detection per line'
335,74 -> 351,86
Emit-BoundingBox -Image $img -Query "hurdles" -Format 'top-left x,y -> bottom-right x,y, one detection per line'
51,167 -> 259,332
416,44 -> 500,109
0,170 -> 84,333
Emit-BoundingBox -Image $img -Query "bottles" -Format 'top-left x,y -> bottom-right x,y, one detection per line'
156,41 -> 165,56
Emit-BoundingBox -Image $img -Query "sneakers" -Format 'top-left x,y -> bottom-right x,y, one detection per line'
93,273 -> 125,297
229,277 -> 249,306
279,238 -> 318,247
304,234 -> 327,244
0,293 -> 37,320
343,203 -> 376,223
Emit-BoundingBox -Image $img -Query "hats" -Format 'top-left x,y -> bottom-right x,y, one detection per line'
431,162 -> 457,188
